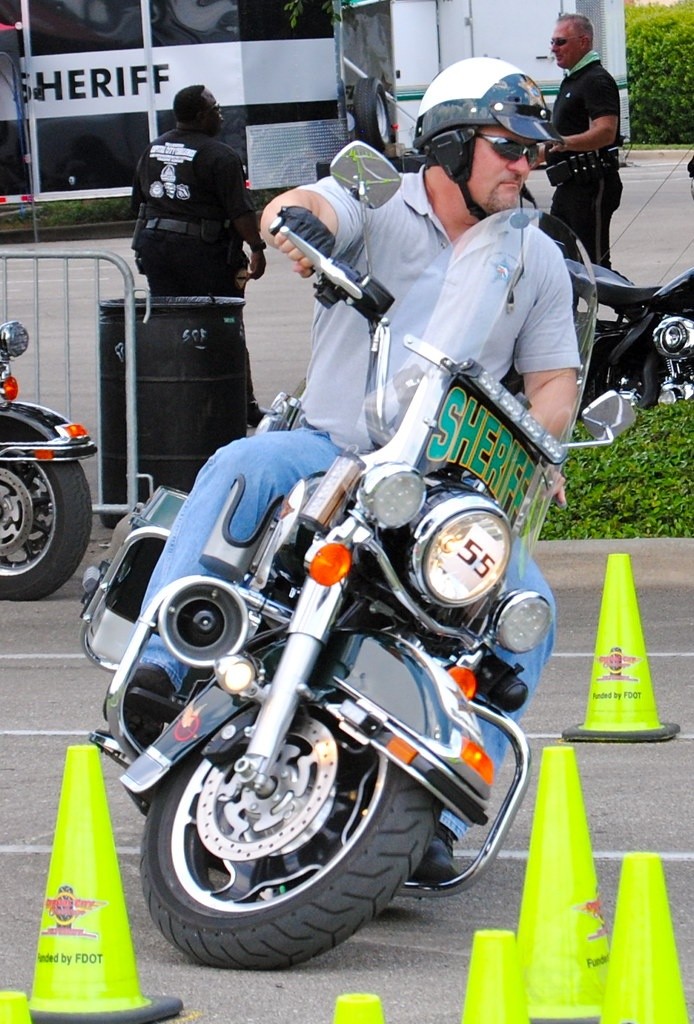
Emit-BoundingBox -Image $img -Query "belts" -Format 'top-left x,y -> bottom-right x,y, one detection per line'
143,219 -> 225,241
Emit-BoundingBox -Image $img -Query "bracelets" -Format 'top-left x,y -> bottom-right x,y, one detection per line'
251,240 -> 268,252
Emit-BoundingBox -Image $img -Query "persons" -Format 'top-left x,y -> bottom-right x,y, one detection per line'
131,84 -> 268,425
104,58 -> 580,889
530,13 -> 634,286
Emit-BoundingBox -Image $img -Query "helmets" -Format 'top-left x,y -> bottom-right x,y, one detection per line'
412,57 -> 566,146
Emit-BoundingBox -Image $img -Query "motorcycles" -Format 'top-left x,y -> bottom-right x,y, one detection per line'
0,320 -> 98,601
82,142 -> 637,970
566,155 -> 694,418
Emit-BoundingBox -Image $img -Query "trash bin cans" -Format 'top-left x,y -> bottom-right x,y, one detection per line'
100,295 -> 246,525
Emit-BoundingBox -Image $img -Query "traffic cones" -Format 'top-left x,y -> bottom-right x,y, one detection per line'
599,851 -> 690,1024
562,554 -> 682,743
517,746 -> 611,1024
332,994 -> 386,1024
462,928 -> 532,1024
0,745 -> 183,1024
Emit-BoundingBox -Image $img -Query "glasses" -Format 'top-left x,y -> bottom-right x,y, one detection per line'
475,131 -> 540,166
551,33 -> 585,46
204,100 -> 222,116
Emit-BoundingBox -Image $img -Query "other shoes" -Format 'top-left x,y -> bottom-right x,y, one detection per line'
102,662 -> 176,749
409,831 -> 466,890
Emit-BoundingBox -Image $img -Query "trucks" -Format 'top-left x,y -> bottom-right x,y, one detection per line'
0,0 -> 631,206
